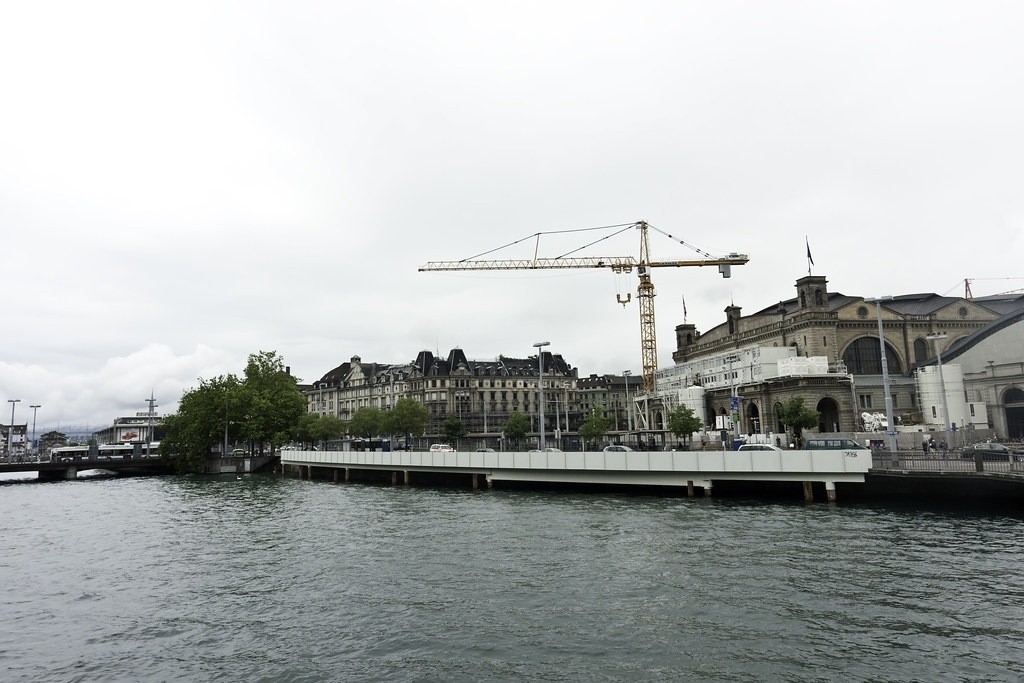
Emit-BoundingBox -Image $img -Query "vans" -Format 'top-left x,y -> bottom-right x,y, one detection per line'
806,438 -> 868,449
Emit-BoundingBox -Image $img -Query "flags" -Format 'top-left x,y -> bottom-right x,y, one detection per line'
807,242 -> 814,266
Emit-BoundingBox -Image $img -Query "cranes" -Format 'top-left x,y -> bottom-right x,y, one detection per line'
419,221 -> 750,393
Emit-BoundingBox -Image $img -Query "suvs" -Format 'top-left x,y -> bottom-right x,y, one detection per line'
430,444 -> 456,452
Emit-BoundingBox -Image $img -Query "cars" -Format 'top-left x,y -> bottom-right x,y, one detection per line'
227,449 -> 250,456
738,444 -> 780,451
603,445 -> 633,452
476,448 -> 494,452
528,448 -> 563,452
263,445 -> 319,454
962,443 -> 1024,462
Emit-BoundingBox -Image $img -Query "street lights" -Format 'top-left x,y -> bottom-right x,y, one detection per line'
8,400 -> 21,465
864,295 -> 899,467
30,405 -> 41,463
564,384 -> 570,431
622,370 -> 632,431
319,383 -> 327,420
533,342 -> 550,452
925,332 -> 955,450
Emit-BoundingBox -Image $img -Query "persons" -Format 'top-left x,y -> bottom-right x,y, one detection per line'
776,435 -> 803,449
922,437 -> 948,455
586,436 -> 707,453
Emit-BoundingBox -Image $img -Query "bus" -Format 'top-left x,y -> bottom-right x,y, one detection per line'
50,441 -> 160,462
